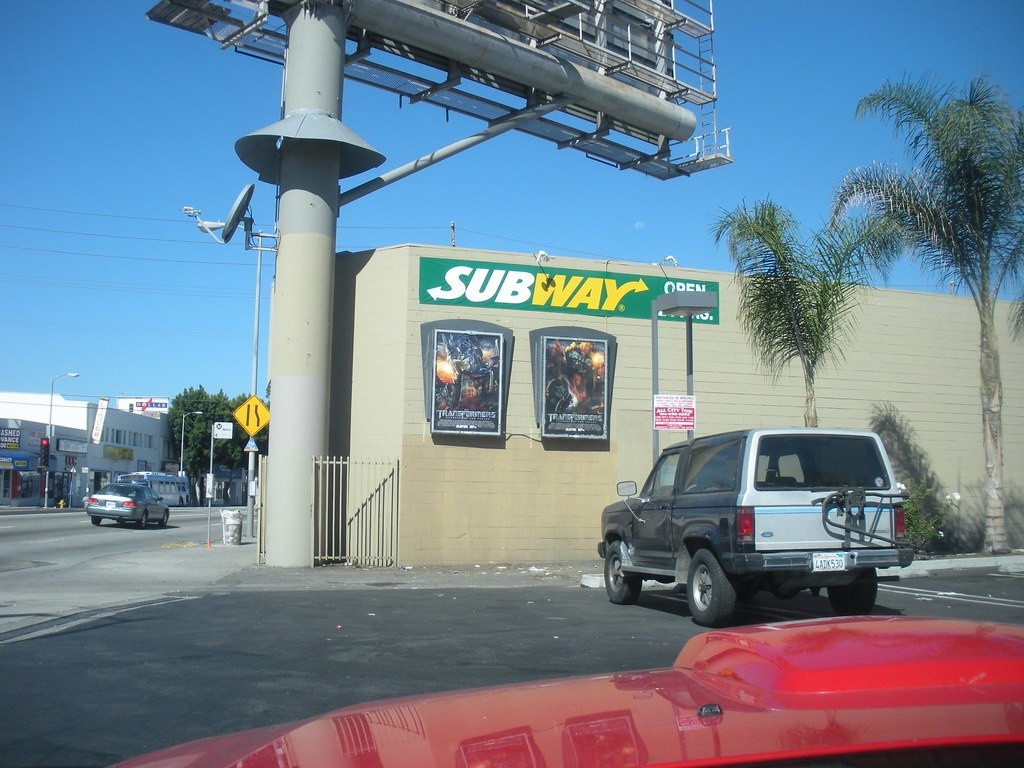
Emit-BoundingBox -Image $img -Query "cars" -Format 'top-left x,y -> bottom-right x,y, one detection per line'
86,483 -> 170,528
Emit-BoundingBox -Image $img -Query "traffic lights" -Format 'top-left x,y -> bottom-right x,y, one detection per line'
40,437 -> 49,466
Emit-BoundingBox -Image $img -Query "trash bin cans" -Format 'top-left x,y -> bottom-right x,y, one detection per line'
222,511 -> 243,546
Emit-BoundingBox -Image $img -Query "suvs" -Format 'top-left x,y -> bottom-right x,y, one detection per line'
596,427 -> 916,628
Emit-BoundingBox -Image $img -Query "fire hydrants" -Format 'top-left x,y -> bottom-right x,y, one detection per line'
57,499 -> 67,509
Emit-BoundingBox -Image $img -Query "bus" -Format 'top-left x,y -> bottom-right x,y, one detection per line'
116,471 -> 190,507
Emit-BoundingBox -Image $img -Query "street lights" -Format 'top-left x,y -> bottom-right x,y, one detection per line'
180,411 -> 203,477
197,219 -> 263,543
43,373 -> 80,508
649,292 -> 720,469
